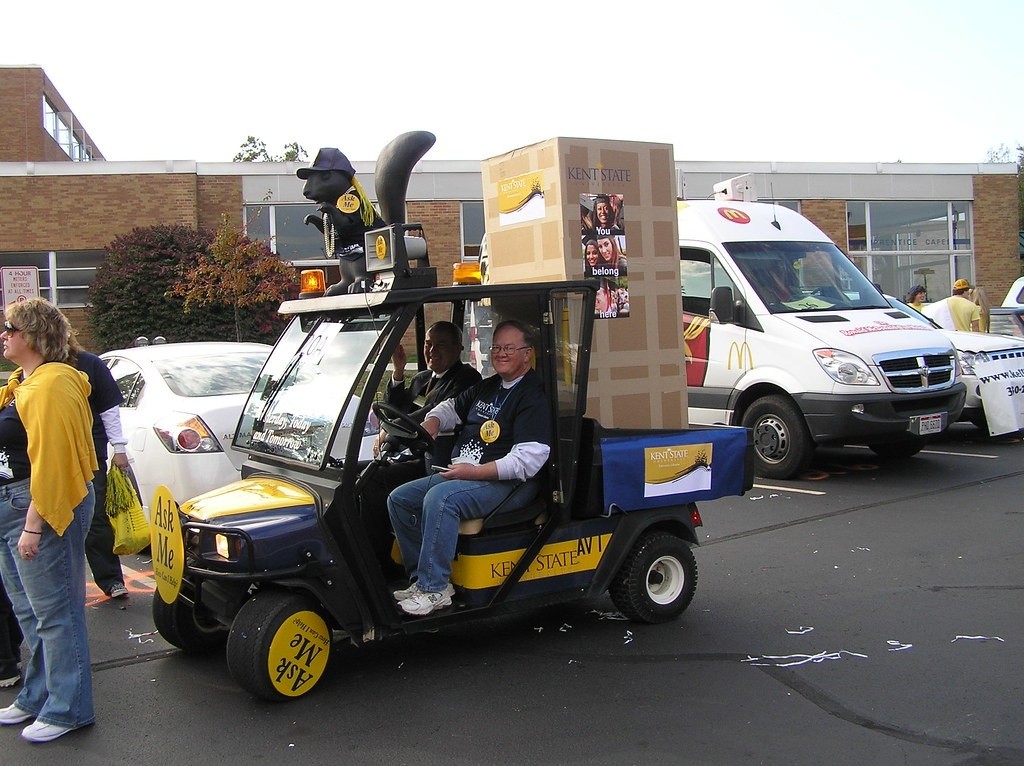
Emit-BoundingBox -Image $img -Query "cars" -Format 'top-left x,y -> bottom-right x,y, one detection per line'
96,341 -> 391,523
986,306 -> 1024,338
802,289 -> 1024,432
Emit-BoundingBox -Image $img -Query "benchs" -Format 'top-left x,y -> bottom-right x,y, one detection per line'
381,422 -> 556,535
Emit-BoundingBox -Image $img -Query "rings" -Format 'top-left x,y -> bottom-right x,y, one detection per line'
25,552 -> 29,556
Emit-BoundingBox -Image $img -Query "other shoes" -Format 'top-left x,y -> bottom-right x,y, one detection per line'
110,583 -> 129,598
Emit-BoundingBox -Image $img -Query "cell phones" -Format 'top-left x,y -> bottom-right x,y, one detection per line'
431,465 -> 450,472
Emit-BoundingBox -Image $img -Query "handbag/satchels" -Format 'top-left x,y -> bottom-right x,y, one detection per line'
101,463 -> 152,556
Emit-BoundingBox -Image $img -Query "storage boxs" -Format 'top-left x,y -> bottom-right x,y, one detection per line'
482,138 -> 688,429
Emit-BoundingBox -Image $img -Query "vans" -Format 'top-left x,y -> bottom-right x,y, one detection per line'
1000,277 -> 1024,307
675,199 -> 968,481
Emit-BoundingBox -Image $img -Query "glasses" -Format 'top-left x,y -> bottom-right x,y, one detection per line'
489,345 -> 533,355
4,320 -> 24,337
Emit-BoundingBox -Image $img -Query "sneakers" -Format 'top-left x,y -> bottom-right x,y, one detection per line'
22,719 -> 85,742
396,588 -> 452,615
0,702 -> 33,723
0,669 -> 21,687
392,577 -> 455,601
14,654 -> 23,670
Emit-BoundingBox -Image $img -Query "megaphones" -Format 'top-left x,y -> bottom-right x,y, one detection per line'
714,173 -> 757,202
364,226 -> 427,272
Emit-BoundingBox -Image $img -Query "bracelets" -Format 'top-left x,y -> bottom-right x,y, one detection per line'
23,529 -> 42,536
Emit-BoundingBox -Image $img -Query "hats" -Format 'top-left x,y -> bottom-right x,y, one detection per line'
953,278 -> 970,290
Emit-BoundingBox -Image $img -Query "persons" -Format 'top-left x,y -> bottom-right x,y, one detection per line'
348,321 -> 483,572
387,320 -> 557,617
902,277 -> 990,333
0,298 -> 129,744
580,193 -> 631,319
